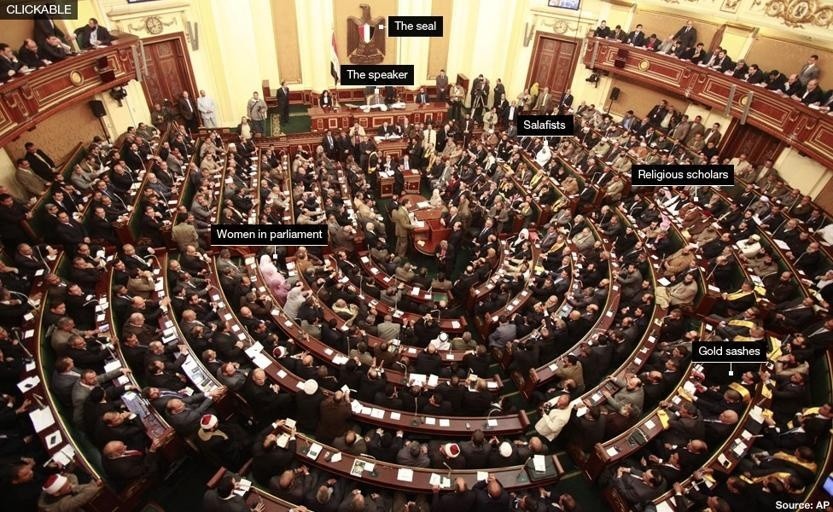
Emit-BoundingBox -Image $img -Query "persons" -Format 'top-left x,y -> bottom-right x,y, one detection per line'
4,18 -> 831,512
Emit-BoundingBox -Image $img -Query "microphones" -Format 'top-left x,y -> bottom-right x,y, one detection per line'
42,449 -> 80,474
13,329 -> 34,365
95,340 -> 119,365
31,392 -> 47,411
35,245 -> 51,279
82,298 -> 107,316
9,290 -> 41,310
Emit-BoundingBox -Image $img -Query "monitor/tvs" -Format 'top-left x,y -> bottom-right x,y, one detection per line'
548,0 -> 580,10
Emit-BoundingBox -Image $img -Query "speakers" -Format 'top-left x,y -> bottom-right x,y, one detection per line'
618,49 -> 628,58
90,100 -> 106,118
610,88 -> 620,100
97,56 -> 108,68
102,70 -> 116,83
615,60 -> 625,68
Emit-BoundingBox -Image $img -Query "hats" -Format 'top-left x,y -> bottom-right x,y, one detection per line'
304,379 -> 318,395
438,333 -> 447,343
499,441 -> 512,457
750,233 -> 760,242
445,442 -> 460,458
40,473 -> 67,494
199,413 -> 217,429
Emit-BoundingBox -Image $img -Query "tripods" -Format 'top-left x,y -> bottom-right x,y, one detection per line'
472,95 -> 485,108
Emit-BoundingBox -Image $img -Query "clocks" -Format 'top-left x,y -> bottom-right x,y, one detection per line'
553,20 -> 568,34
146,16 -> 163,34
